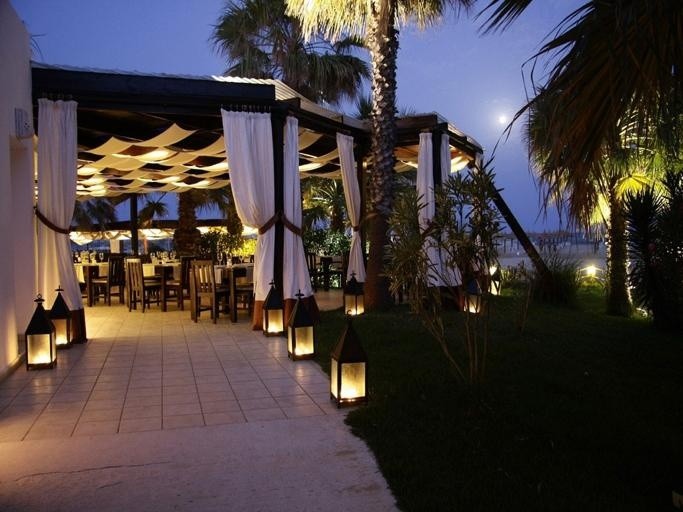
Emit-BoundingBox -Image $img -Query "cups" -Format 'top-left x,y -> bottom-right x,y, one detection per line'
150,250 -> 177,264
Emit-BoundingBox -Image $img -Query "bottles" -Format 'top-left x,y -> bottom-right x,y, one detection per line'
226,259 -> 232,268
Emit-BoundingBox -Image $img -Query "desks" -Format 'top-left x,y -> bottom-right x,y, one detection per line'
73,264 -> 93,307
308,256 -> 343,292
187,263 -> 253,324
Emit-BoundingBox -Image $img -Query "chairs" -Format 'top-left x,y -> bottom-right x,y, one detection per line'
305,251 -> 321,293
92,251 -> 227,327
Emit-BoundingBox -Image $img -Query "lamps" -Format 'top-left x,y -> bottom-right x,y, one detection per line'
261,272 -> 370,409
24,285 -> 74,371
462,266 -> 503,314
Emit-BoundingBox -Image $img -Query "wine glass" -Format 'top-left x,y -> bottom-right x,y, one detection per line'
239,252 -> 244,264
217,252 -> 223,266
98,253 -> 105,263
